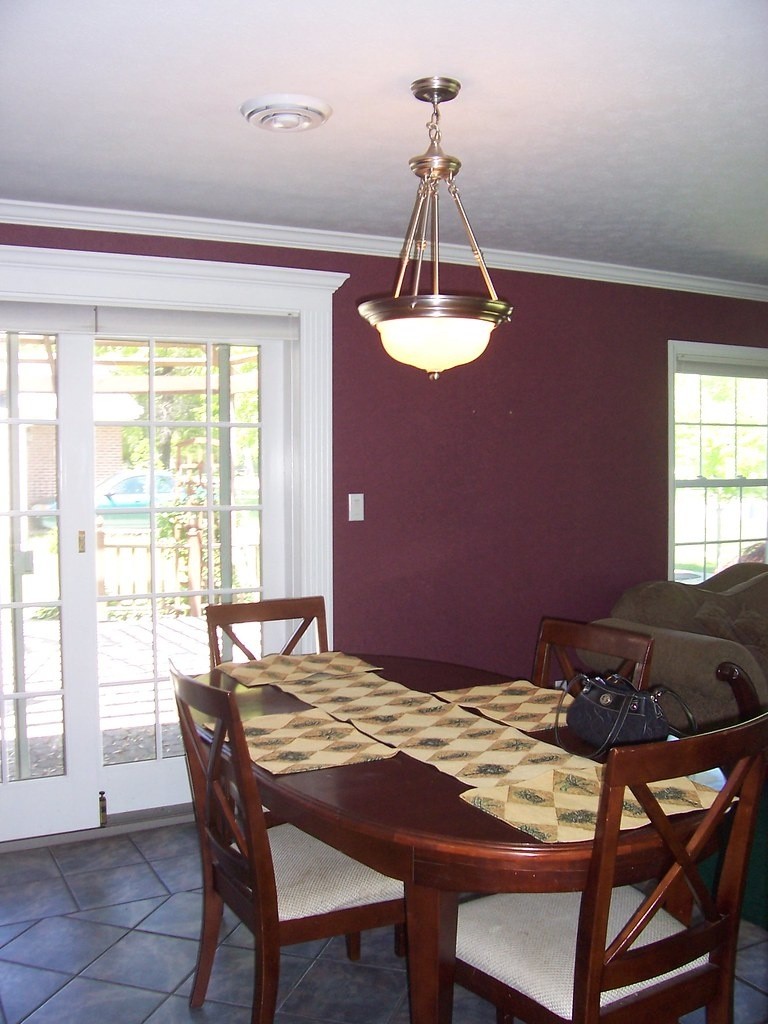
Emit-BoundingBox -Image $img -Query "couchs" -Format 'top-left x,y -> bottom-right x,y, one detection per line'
575,562 -> 768,735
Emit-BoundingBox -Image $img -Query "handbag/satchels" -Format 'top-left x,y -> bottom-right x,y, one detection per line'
555,674 -> 697,758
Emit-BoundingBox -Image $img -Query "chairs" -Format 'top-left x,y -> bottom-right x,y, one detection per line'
167,656 -> 408,1024
454,710 -> 768,1024
206,595 -> 330,830
531,616 -> 655,692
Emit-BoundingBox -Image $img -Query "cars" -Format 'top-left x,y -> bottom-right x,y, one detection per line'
29,469 -> 215,530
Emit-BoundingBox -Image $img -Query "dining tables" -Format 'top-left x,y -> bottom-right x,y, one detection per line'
188,655 -> 736,1023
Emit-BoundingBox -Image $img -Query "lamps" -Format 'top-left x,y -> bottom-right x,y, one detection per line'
238,93 -> 332,135
356,78 -> 514,382
